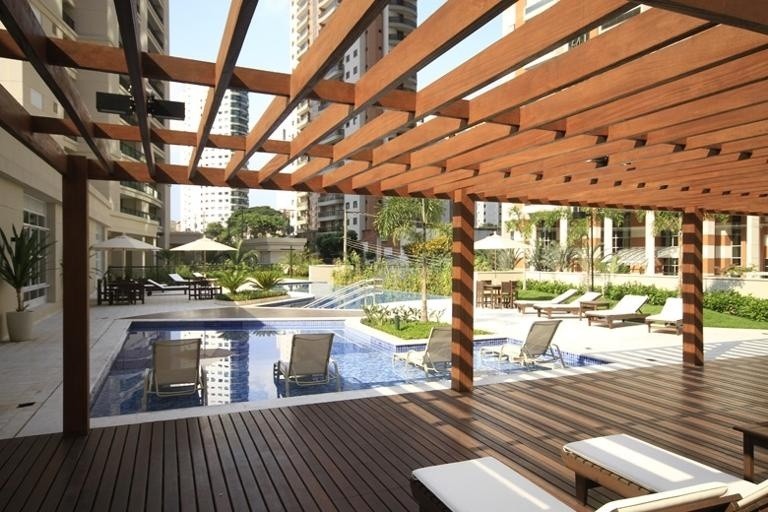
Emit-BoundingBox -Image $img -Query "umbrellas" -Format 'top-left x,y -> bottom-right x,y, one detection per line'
89,232 -> 164,302
169,234 -> 238,298
473,231 -> 531,304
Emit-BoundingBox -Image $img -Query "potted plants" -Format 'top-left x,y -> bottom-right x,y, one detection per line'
0,222 -> 58,341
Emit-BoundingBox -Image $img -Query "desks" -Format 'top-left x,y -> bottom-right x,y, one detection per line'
580,301 -> 609,321
733,423 -> 768,481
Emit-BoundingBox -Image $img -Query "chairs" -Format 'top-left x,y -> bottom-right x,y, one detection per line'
482,319 -> 567,370
645,297 -> 682,335
140,337 -> 208,412
97,269 -> 224,305
514,288 -> 578,313
533,291 -> 603,318
273,332 -> 340,396
478,280 -> 518,309
392,326 -> 452,380
565,432 -> 767,511
405,451 -> 743,512
584,294 -> 651,329
